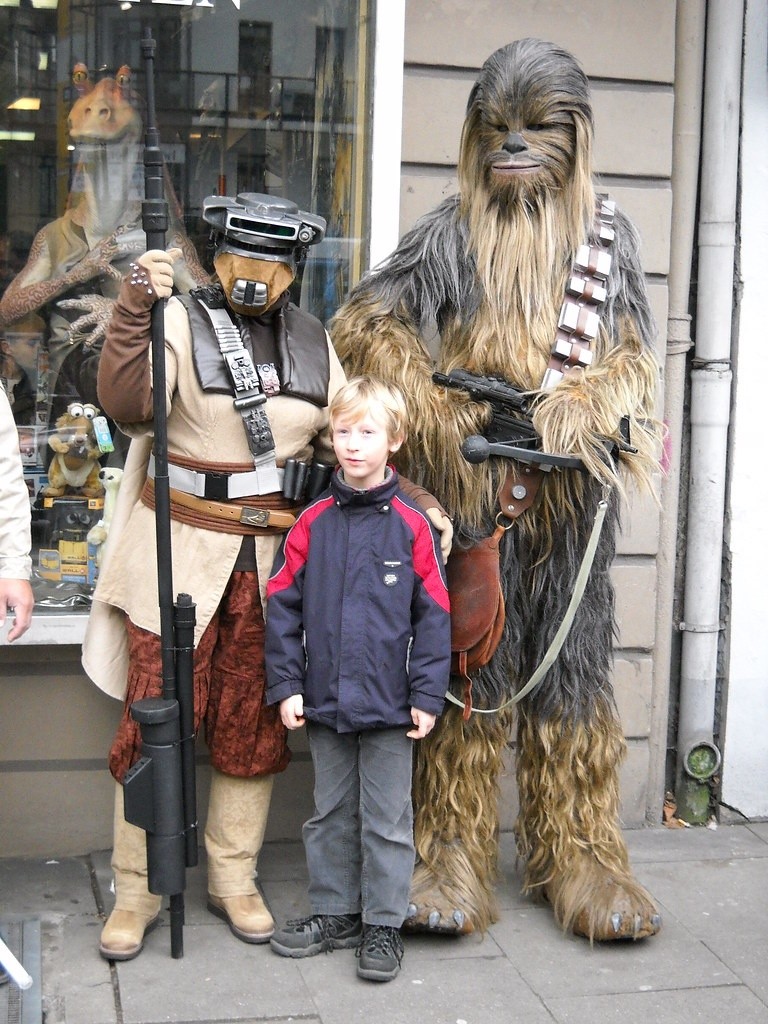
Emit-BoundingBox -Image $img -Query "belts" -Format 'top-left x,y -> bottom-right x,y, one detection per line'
145,474 -> 302,527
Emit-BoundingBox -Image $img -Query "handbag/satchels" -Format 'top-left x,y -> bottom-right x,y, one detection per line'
439,531 -> 506,676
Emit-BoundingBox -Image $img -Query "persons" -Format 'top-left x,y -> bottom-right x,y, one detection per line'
0,229 -> 46,427
264,376 -> 451,981
82,190 -> 455,961
0,380 -> 34,984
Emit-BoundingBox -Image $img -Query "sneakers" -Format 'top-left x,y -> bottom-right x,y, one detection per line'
271,914 -> 365,958
355,923 -> 406,980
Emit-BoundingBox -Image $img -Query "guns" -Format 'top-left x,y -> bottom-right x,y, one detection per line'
431,366 -> 640,468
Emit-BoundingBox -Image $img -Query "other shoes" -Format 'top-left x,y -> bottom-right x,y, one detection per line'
207,891 -> 277,943
99,907 -> 159,961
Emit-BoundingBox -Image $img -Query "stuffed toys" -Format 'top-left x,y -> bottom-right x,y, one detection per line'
327,39 -> 663,944
